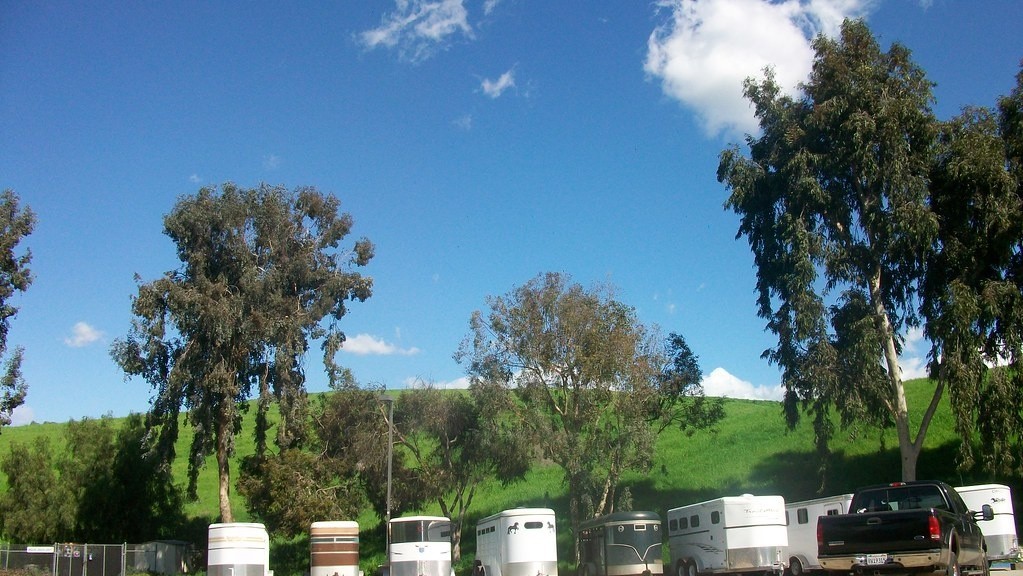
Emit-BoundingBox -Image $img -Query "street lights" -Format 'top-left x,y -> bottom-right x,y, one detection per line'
378,393 -> 395,560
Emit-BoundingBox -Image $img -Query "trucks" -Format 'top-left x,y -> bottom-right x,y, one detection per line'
472,508 -> 559,576
207,522 -> 270,576
901,484 -> 1019,568
667,492 -> 790,576
576,510 -> 664,576
784,493 -> 856,576
309,521 -> 360,576
389,516 -> 452,576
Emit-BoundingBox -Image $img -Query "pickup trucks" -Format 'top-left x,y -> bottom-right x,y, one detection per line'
817,480 -> 994,576
